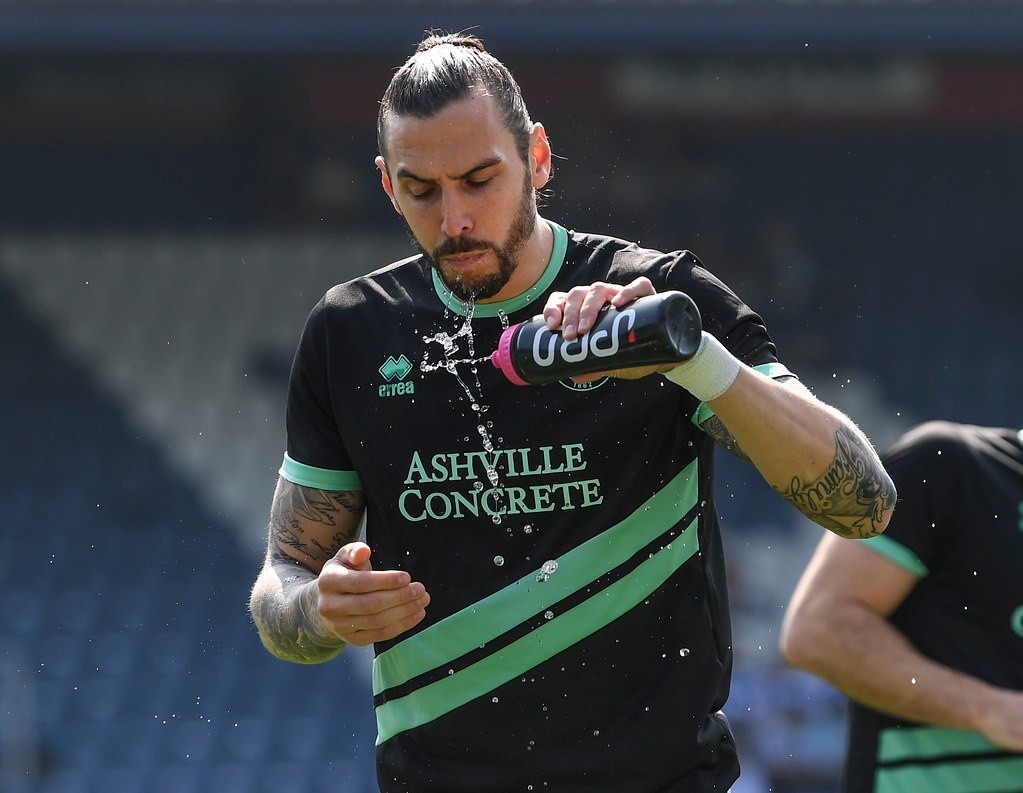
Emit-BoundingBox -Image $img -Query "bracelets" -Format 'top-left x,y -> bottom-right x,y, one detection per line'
662,331 -> 741,402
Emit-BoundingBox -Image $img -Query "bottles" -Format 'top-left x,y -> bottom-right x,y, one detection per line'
489,291 -> 702,385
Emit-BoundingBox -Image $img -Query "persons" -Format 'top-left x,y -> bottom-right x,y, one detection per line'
250,35 -> 897,793
783,420 -> 1023,793
